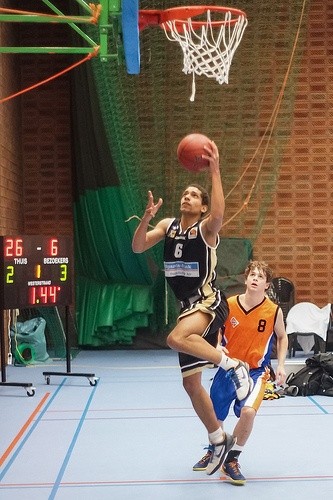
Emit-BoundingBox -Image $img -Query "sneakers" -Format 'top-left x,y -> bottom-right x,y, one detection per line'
221,458 -> 246,485
207,432 -> 237,475
226,358 -> 254,401
193,446 -> 216,471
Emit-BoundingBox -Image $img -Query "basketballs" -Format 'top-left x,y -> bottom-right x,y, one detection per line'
177,133 -> 212,172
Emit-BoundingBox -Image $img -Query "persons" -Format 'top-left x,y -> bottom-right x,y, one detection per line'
193,261 -> 289,485
131,140 -> 255,475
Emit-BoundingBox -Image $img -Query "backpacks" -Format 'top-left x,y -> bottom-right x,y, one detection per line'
289,352 -> 333,397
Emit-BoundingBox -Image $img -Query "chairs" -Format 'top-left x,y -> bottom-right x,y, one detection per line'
272,277 -> 333,359
